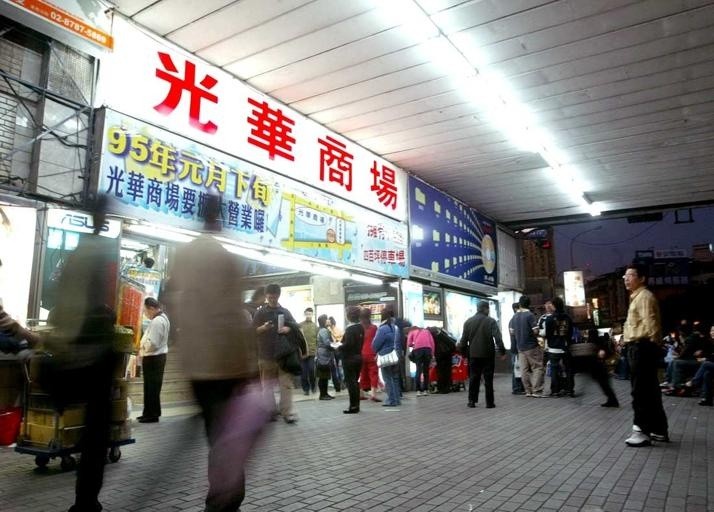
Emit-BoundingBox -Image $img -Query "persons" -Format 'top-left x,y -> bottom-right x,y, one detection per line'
428,326 -> 456,394
252,283 -> 299,422
317,314 -> 334,400
334,307 -> 364,413
0,310 -> 39,344
537,298 -> 629,407
358,308 -> 382,403
137,297 -> 170,423
298,308 -> 319,395
372,310 -> 403,406
623,267 -> 670,446
462,301 -> 507,408
509,304 -> 526,394
407,326 -> 434,395
660,319 -> 714,405
243,286 -> 266,383
512,295 -> 546,397
58,192 -> 118,512
164,196 -> 254,512
327,317 -> 345,389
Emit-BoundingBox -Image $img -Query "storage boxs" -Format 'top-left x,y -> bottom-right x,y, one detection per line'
20,319 -> 137,450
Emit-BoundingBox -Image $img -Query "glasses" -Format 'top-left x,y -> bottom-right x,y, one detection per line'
622,274 -> 643,279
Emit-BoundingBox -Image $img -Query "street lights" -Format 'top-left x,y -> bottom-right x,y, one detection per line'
567,223 -> 604,270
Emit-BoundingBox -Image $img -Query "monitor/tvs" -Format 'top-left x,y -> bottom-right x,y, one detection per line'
423,286 -> 443,320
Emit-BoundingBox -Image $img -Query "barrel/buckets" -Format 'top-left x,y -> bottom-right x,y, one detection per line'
1,404 -> 22,446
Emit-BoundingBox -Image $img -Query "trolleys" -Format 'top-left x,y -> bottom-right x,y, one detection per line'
6,345 -> 138,472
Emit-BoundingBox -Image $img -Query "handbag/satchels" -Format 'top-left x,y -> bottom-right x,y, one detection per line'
376,349 -> 399,368
459,344 -> 471,359
276,342 -> 305,376
514,354 -> 522,379
407,351 -> 416,364
314,362 -> 332,381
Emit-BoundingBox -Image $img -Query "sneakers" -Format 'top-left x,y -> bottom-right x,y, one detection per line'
625,425 -> 651,447
511,389 -> 575,398
601,398 -> 619,408
343,407 -> 360,414
269,412 -> 297,424
468,403 -> 496,408
650,431 -> 670,442
136,413 -> 159,423
302,388 -> 451,406
